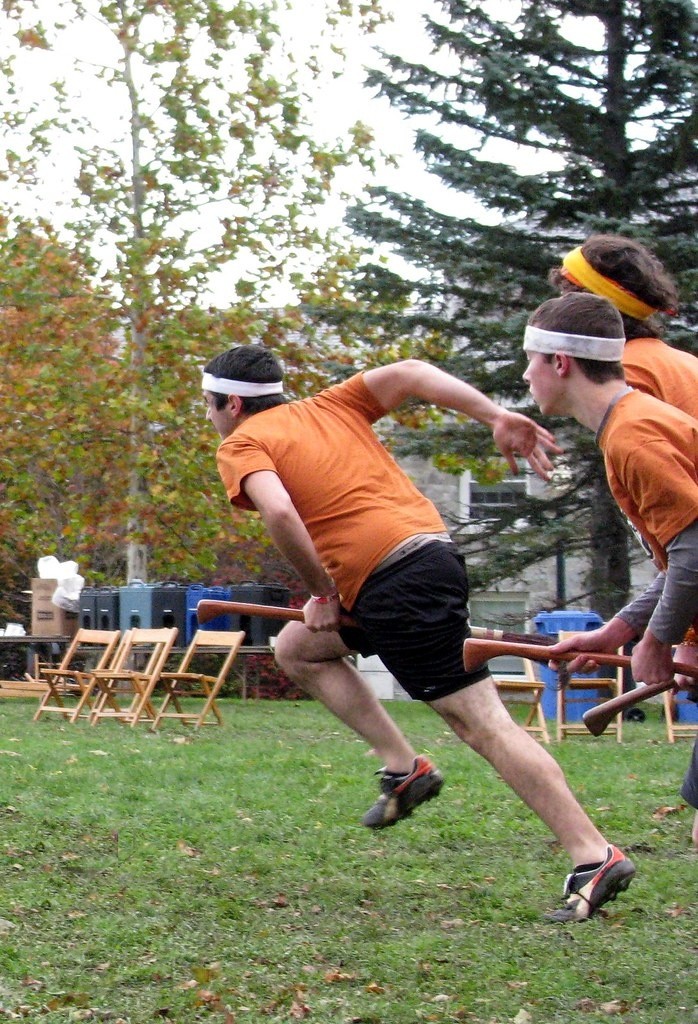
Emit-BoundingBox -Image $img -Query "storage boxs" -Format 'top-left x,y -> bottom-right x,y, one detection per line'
21,577 -> 80,638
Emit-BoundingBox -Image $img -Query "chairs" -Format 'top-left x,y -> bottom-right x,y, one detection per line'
40,626 -> 245,745
490,631 -> 698,745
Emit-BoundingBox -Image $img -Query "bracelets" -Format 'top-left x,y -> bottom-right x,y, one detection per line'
311,585 -> 338,604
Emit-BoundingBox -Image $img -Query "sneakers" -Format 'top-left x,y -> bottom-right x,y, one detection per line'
544,844 -> 635,924
362,754 -> 444,830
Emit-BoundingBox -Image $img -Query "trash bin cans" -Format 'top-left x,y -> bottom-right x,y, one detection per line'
151,580 -> 205,649
229,580 -> 291,647
118,580 -> 160,645
78,585 -> 120,647
531,607 -> 697,726
185,583 -> 232,648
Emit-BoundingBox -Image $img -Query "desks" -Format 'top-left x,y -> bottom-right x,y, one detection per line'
0,636 -> 276,705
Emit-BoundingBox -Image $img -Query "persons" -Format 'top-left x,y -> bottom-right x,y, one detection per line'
523,294 -> 698,847
549,235 -> 698,695
201,345 -> 637,923
